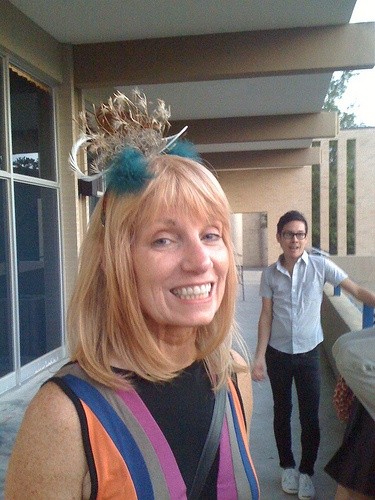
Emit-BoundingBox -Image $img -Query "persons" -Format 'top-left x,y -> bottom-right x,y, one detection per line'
253,211 -> 375,500
5,91 -> 260,500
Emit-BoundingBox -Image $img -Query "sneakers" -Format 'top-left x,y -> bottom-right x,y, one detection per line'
297,473 -> 315,500
280,467 -> 299,495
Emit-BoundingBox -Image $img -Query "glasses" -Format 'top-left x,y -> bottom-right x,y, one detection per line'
279,231 -> 307,240
